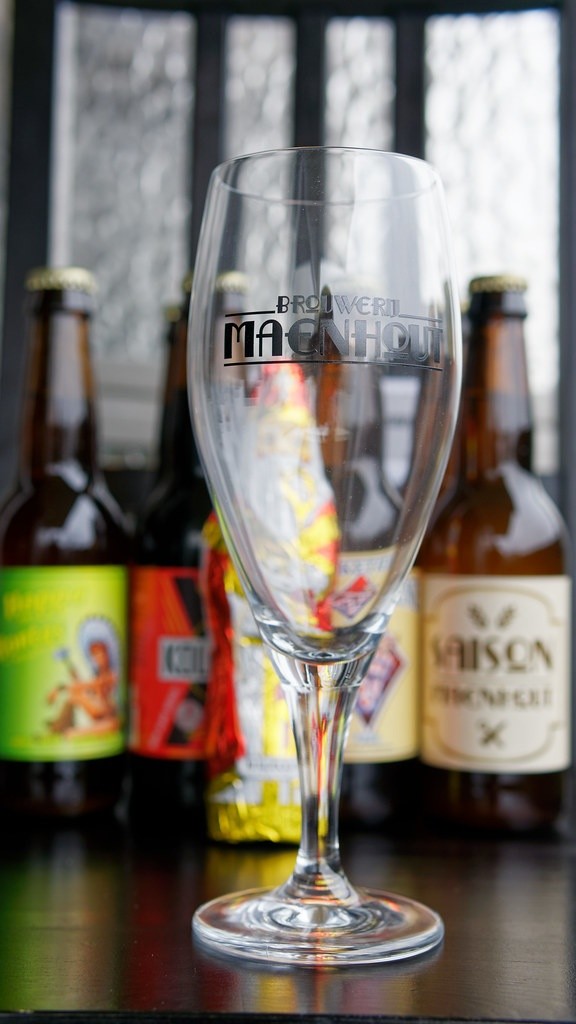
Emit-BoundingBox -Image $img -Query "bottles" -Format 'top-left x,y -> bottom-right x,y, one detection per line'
201,360 -> 343,848
420,266 -> 572,841
0,268 -> 132,842
132,304 -> 214,835
315,277 -> 420,829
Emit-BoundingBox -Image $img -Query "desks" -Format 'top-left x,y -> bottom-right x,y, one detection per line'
0,790 -> 576,1024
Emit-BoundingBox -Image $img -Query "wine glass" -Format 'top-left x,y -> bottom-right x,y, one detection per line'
188,151 -> 464,968
